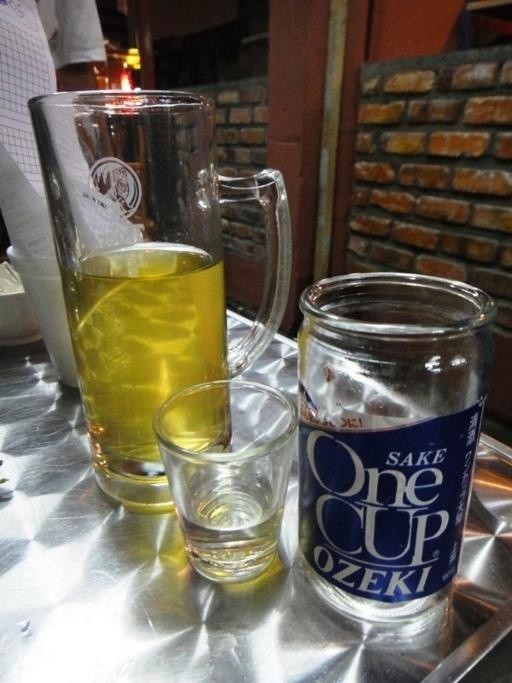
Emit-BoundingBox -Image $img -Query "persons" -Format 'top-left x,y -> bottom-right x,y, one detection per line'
1,0 -> 108,257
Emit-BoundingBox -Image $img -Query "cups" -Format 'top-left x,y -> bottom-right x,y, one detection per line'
28,253 -> 81,391
26,93 -> 294,510
152,380 -> 300,582
300,272 -> 501,624
